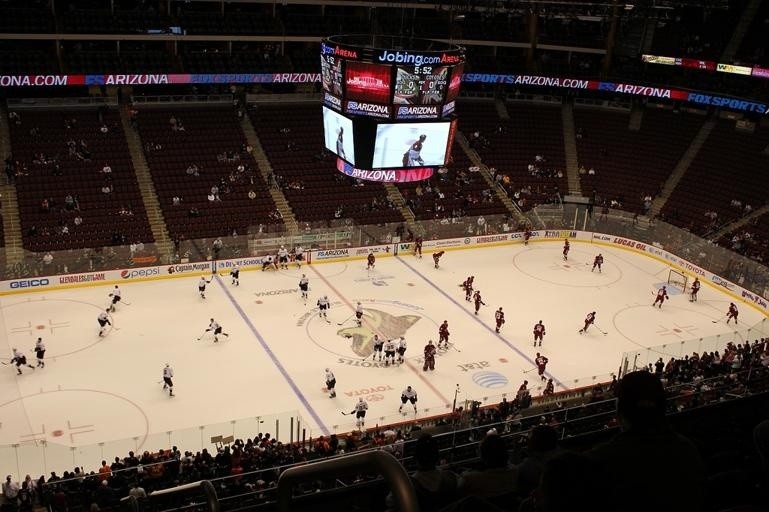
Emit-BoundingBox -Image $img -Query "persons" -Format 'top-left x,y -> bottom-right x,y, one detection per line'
0,88 -> 768,508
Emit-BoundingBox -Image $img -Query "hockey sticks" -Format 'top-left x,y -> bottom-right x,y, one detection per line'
220,274 -> 230,277
522,369 -> 536,373
118,301 -> 131,305
341,412 -> 351,415
712,316 -> 727,323
1,361 -> 12,365
593,324 -> 608,335
586,261 -> 594,265
652,291 -> 666,297
198,331 -> 206,340
337,313 -> 356,325
206,276 -> 213,286
110,325 -> 121,330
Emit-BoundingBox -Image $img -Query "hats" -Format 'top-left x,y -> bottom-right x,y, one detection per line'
613,371 -> 666,423
